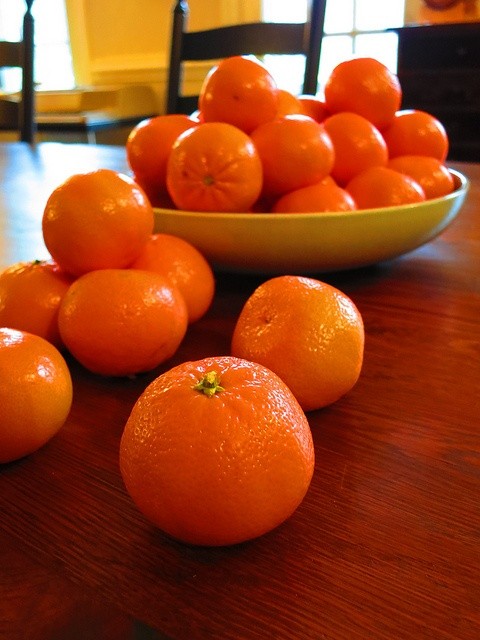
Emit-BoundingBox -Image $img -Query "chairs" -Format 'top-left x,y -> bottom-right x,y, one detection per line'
160,0 -> 327,117
0,0 -> 39,142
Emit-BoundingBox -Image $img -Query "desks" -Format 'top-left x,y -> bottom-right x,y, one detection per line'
0,90 -> 159,145
0,140 -> 480,640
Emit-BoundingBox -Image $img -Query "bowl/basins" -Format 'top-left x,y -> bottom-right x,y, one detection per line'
151,167 -> 471,277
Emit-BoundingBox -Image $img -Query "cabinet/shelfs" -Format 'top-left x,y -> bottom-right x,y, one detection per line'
386,22 -> 479,163
